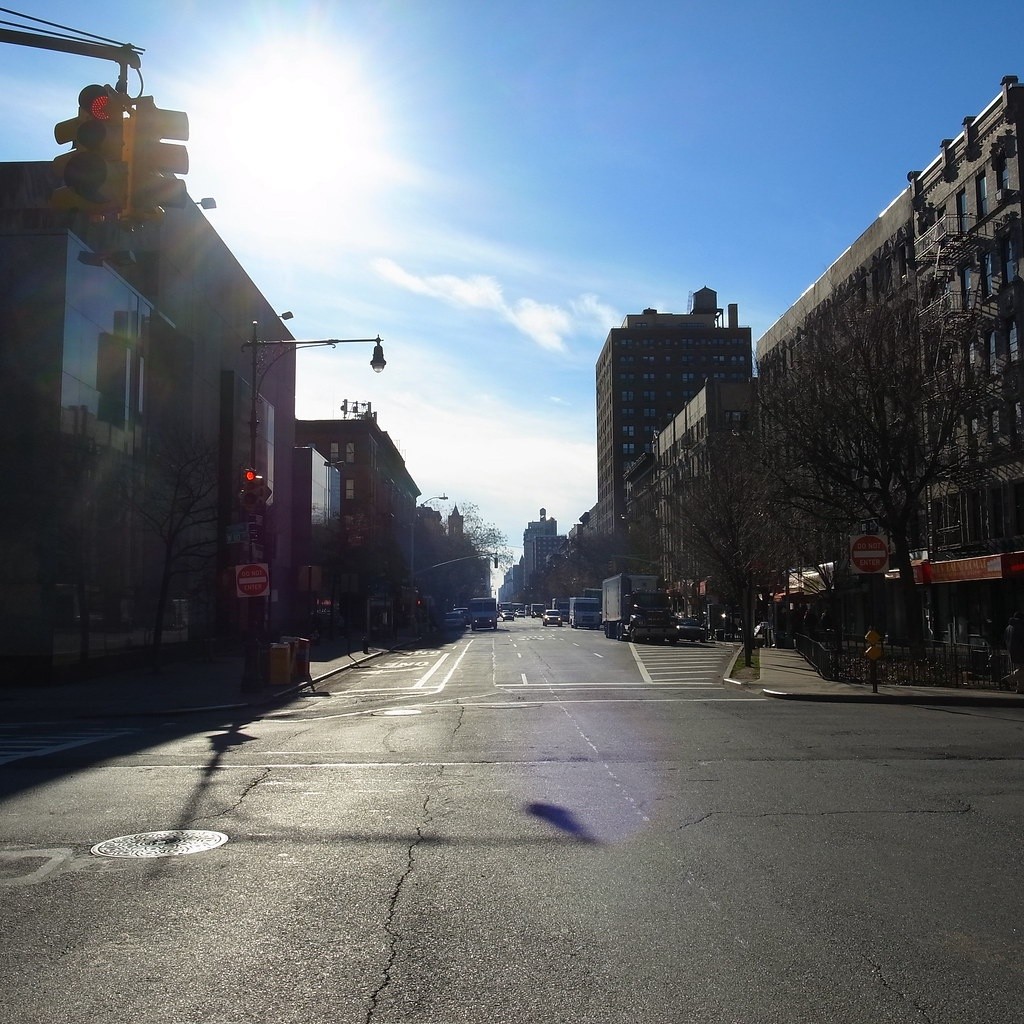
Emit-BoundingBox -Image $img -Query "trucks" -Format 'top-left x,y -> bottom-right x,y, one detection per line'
602,573 -> 679,646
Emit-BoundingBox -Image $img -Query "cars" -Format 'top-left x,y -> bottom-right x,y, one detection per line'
542,609 -> 562,627
443,611 -> 466,630
498,602 -> 545,620
676,618 -> 707,643
453,608 -> 471,626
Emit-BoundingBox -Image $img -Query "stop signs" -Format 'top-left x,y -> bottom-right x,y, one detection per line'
850,535 -> 889,573
235,563 -> 270,598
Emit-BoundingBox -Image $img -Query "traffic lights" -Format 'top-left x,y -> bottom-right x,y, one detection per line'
124,94 -> 188,225
240,469 -> 264,517
52,83 -> 125,224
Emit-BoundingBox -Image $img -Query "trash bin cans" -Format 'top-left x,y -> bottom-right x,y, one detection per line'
298,638 -> 311,678
270,643 -> 291,686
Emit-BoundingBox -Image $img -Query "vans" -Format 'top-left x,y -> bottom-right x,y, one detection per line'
469,597 -> 498,631
569,597 -> 601,630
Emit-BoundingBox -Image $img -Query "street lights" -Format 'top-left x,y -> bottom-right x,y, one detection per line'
238,320 -> 387,691
409,495 -> 448,641
